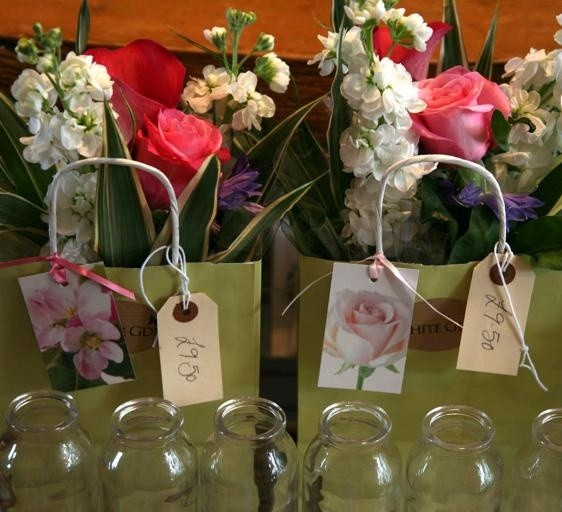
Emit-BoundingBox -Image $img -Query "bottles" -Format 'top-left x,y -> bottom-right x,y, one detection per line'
404,404 -> 505,510
0,388 -> 104,511
196,395 -> 300,512
516,403 -> 562,512
98,395 -> 201,511
299,398 -> 409,512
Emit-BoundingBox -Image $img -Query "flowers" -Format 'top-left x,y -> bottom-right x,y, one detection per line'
260,0 -> 562,265
0,0 -> 329,268
